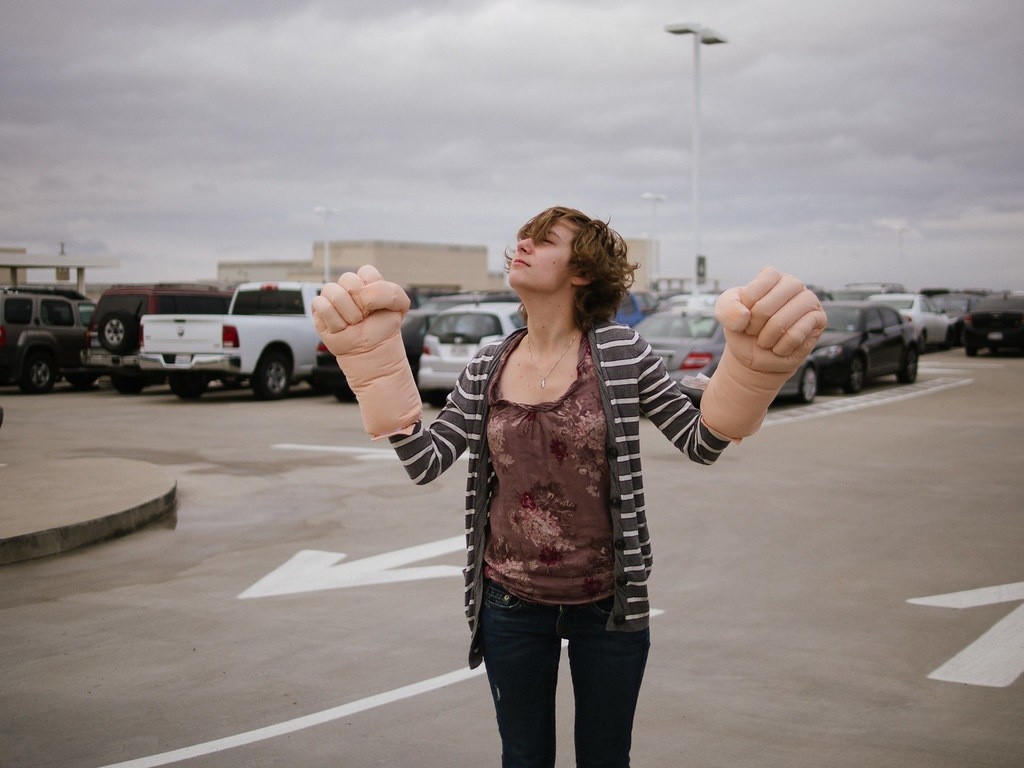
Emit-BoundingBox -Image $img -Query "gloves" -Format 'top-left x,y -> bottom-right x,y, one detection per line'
701,268 -> 827,444
312,265 -> 423,440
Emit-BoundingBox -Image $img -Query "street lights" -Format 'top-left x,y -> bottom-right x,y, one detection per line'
665,22 -> 730,288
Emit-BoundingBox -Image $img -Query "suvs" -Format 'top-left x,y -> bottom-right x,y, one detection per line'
0,280 -> 97,395
79,284 -> 234,393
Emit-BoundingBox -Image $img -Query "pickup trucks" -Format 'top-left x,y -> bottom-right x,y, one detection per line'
137,281 -> 332,400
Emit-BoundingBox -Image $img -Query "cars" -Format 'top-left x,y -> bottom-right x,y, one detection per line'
810,299 -> 920,395
864,293 -> 952,354
927,291 -> 978,320
802,283 -> 906,301
962,293 -> 1024,357
416,301 -> 528,410
308,291 -> 520,403
610,292 -> 718,328
631,308 -> 818,407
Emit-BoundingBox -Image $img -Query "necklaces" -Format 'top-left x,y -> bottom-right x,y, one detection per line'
529,335 -> 577,389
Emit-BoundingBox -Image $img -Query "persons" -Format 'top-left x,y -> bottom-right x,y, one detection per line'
313,206 -> 828,768
